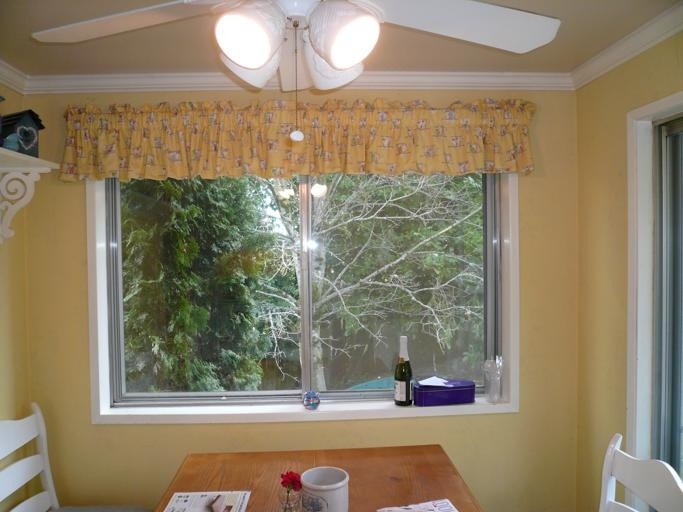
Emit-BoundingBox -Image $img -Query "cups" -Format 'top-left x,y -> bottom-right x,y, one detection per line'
479,355 -> 504,405
300,465 -> 349,512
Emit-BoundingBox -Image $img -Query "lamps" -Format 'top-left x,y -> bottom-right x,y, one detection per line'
213,0 -> 380,91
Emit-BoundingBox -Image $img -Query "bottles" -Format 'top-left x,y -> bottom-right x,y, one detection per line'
393,335 -> 414,406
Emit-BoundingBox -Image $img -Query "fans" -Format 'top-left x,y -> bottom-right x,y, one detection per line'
31,0 -> 561,91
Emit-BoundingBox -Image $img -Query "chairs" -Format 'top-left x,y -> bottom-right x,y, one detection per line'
597,434 -> 682,512
0,401 -> 152,512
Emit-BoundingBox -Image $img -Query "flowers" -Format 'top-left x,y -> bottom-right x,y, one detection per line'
279,470 -> 301,512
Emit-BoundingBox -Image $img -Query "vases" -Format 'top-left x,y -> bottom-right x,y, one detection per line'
277,489 -> 301,512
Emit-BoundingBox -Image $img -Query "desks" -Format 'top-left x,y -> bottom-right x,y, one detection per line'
155,444 -> 484,512
